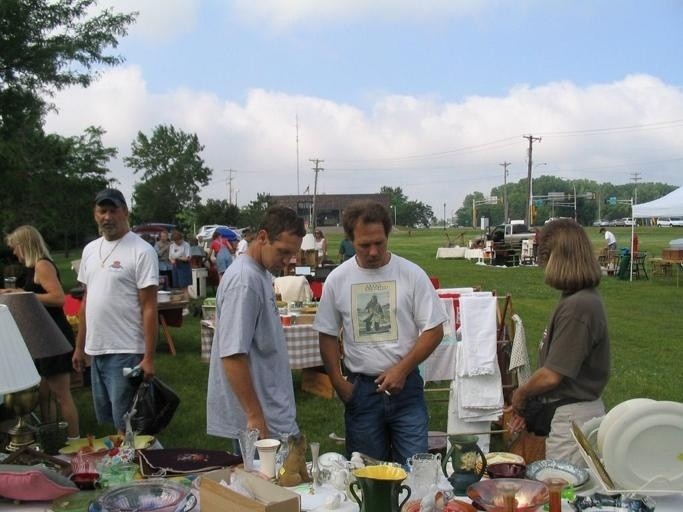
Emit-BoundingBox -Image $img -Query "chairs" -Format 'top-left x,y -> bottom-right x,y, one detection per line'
437,234 -> 683,280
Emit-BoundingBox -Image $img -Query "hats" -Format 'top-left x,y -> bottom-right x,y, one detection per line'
93,188 -> 126,208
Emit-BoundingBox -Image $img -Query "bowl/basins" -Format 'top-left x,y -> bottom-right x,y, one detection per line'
69,471 -> 98,487
91,482 -> 198,511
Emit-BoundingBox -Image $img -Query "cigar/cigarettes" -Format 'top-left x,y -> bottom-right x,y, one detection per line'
377,384 -> 391,396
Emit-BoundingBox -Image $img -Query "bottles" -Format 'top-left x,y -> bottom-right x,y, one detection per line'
405,452 -> 441,500
348,465 -> 409,512
430,277 -> 439,289
309,441 -> 322,491
441,433 -> 486,497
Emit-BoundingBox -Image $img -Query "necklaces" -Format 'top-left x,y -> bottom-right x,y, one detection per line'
98,237 -> 122,267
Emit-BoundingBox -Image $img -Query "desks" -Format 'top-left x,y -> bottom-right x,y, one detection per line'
1,438 -> 682,508
200,278 -> 516,387
157,289 -> 189,353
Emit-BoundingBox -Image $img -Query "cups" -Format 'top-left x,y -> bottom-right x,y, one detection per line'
252,439 -> 280,487
110,462 -> 140,484
4,277 -> 15,290
36,420 -> 69,454
236,426 -> 258,472
276,300 -> 303,327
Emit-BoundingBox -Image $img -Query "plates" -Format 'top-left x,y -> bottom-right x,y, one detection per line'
581,397 -> 683,498
298,306 -> 317,313
465,450 -> 590,511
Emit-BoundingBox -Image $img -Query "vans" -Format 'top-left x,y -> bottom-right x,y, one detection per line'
656,217 -> 682,227
129,222 -> 178,240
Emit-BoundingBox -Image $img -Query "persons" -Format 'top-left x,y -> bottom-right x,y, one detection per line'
599,227 -> 617,266
205,205 -> 306,459
140,228 -> 356,317
503,219 -> 611,464
313,198 -> 448,471
0,226 -> 81,440
72,188 -> 160,432
363,295 -> 384,333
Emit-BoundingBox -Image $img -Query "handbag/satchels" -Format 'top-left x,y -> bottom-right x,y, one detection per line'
523,396 -> 586,437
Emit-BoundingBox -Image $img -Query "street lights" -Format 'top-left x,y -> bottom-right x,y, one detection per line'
390,205 -> 396,228
526,160 -> 550,228
443,203 -> 446,228
560,176 -> 576,224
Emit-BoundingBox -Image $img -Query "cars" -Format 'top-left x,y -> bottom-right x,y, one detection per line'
470,222 -> 538,248
544,217 -> 557,223
196,224 -> 252,240
592,216 -> 637,228
